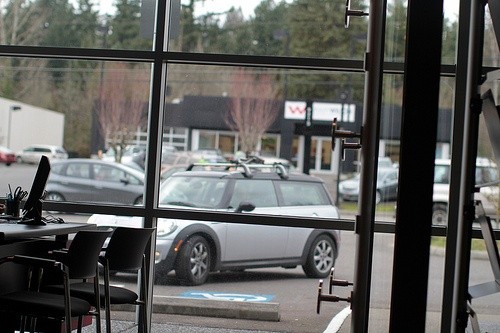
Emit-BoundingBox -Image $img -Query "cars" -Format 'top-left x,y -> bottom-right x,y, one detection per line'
40,158 -> 146,207
431,156 -> 500,225
0,145 -> 69,166
91,145 -> 295,174
338,162 -> 399,204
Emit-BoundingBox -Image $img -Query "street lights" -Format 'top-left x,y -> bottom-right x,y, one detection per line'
7,105 -> 22,148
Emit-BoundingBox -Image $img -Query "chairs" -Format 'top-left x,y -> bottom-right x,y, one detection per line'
0,227 -> 116,333
43,226 -> 157,333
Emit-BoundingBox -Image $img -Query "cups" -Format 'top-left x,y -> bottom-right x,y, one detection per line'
4,199 -> 19,217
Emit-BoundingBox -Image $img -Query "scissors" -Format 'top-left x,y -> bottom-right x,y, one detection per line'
14,186 -> 23,200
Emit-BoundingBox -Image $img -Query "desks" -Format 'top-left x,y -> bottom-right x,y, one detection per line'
0,216 -> 99,333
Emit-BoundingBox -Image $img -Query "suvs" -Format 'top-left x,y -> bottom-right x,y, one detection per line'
88,163 -> 340,286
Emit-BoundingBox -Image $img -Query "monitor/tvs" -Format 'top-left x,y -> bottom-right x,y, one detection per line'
17,154 -> 51,227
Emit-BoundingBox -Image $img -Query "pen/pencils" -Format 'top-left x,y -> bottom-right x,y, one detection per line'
18,190 -> 28,199
8,184 -> 12,195
